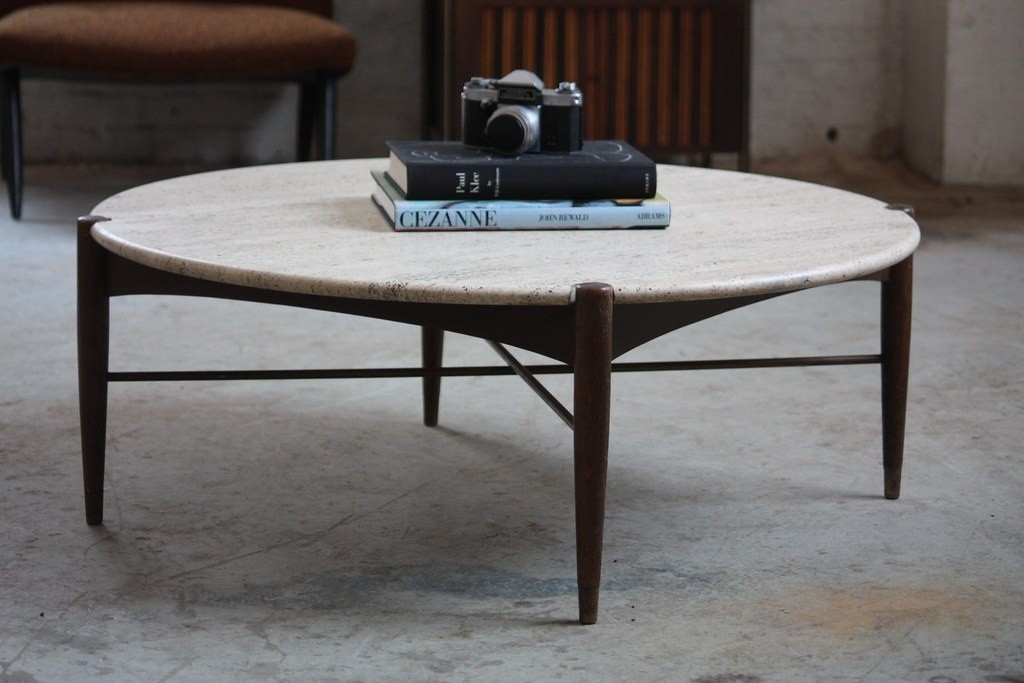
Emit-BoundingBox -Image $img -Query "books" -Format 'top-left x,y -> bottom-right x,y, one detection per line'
383,142 -> 657,200
370,168 -> 673,229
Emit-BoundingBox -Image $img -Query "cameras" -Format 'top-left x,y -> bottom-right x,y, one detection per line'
461,70 -> 584,156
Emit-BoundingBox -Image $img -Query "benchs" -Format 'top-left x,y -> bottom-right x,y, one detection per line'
0,2 -> 358,219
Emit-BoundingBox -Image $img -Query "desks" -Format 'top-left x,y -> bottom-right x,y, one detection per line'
75,157 -> 921,626
